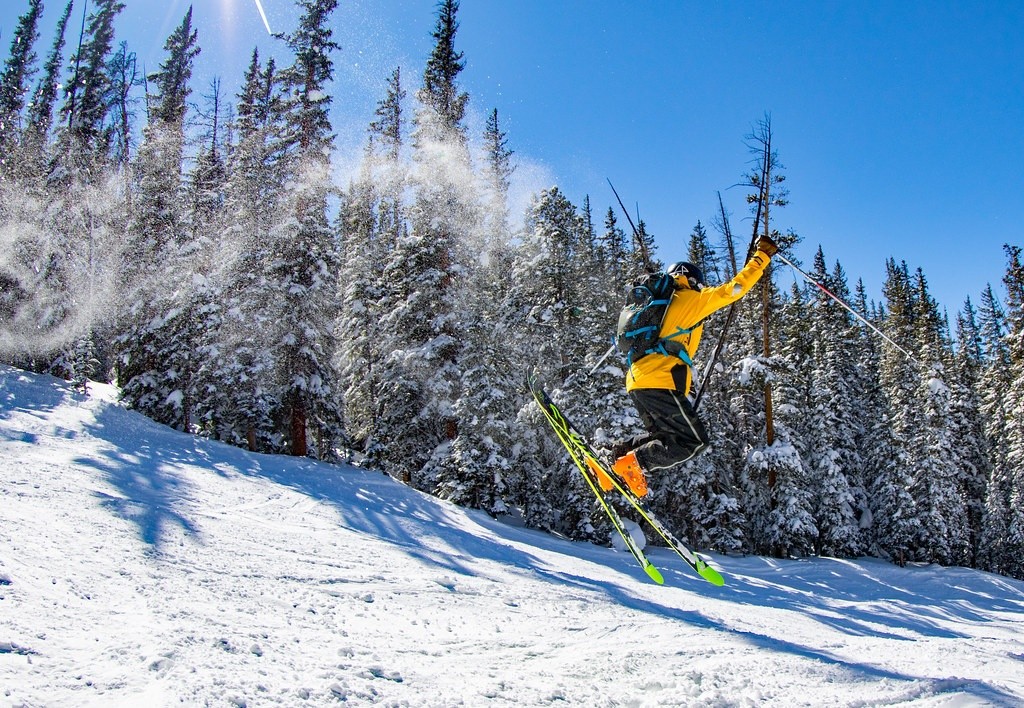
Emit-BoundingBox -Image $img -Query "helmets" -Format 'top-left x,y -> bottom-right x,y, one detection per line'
667,262 -> 705,292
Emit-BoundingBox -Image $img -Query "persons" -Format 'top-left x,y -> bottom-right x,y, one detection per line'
585,233 -> 781,494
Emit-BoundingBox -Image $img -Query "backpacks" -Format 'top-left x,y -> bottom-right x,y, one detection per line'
610,272 -> 694,369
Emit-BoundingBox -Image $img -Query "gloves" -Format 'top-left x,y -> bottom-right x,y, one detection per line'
754,235 -> 777,257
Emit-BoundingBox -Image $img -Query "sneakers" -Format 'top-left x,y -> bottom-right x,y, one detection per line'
614,452 -> 647,497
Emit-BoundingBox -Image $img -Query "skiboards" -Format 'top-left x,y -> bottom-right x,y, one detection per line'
524,362 -> 727,589
